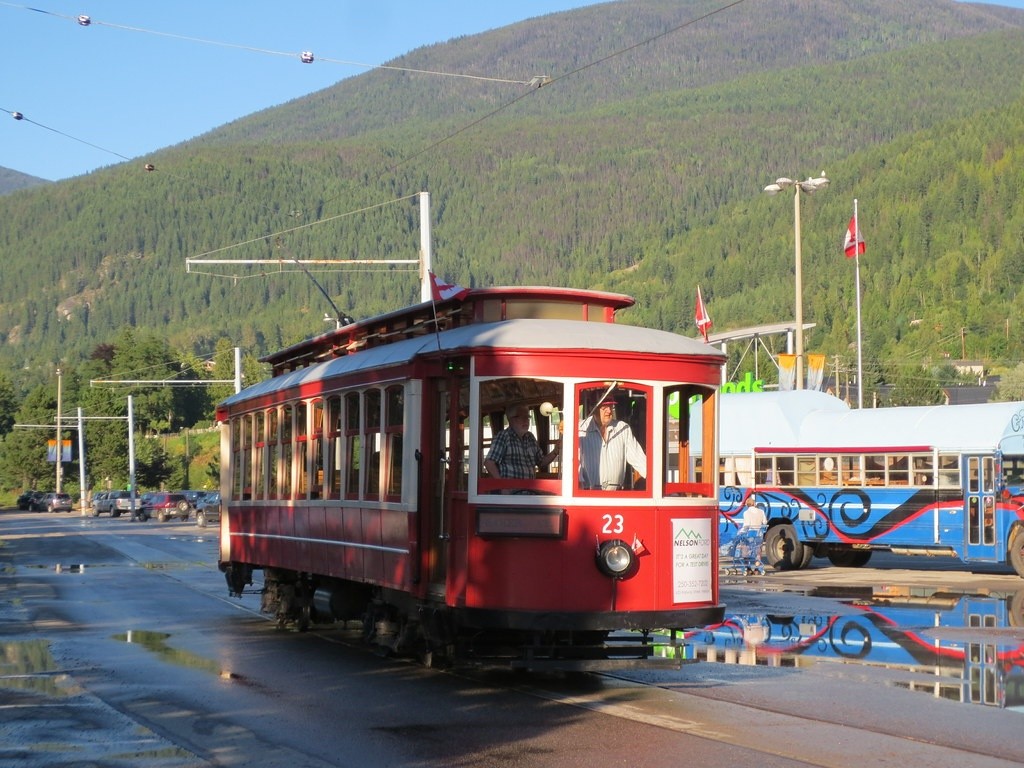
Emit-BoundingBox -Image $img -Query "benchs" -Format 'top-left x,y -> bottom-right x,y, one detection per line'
302,471 -> 383,495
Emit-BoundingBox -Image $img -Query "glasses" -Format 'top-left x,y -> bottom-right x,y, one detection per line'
509,416 -> 532,421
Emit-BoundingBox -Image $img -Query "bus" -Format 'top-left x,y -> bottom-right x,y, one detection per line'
214,258 -> 730,666
667,443 -> 1024,576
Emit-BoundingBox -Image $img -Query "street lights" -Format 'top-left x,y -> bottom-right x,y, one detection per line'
763,169 -> 832,392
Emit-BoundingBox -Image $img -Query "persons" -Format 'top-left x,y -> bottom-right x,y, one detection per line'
760,463 -> 781,485
557,391 -> 647,491
484,401 -> 561,495
739,498 -> 767,575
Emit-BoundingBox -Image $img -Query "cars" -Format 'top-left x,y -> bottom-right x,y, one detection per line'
196,492 -> 221,527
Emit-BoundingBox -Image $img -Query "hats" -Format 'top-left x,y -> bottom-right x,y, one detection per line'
591,390 -> 618,405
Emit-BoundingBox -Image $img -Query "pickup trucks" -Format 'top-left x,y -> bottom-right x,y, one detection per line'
92,490 -> 142,518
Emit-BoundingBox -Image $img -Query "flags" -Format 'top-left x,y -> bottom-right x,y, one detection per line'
696,291 -> 712,342
430,272 -> 469,301
844,215 -> 865,258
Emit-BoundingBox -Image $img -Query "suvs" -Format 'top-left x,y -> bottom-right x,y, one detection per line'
177,490 -> 206,508
17,490 -> 45,512
38,493 -> 73,513
138,492 -> 190,523
